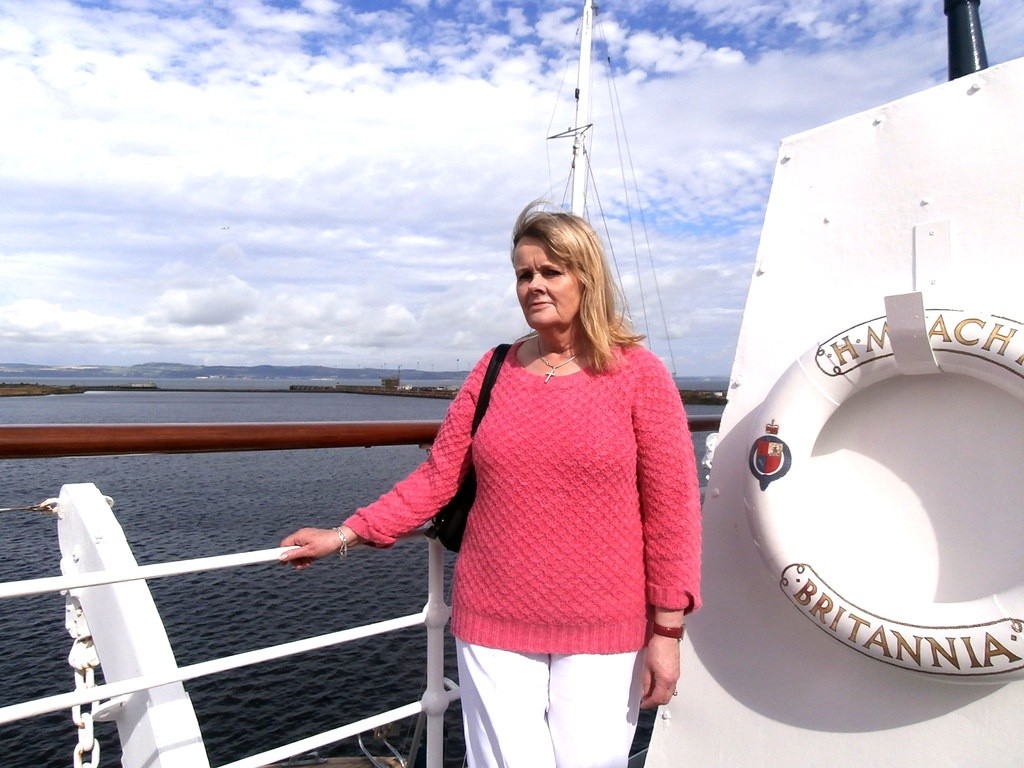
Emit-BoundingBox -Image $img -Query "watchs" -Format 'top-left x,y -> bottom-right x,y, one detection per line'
652,621 -> 686,641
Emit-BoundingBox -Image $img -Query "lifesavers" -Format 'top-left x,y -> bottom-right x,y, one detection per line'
741,304 -> 1024,687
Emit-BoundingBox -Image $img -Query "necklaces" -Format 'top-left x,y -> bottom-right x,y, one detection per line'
537,338 -> 584,383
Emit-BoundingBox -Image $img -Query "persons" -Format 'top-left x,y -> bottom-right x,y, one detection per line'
278,197 -> 701,768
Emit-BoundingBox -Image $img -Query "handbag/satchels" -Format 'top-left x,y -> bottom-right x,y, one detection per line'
421,341 -> 512,554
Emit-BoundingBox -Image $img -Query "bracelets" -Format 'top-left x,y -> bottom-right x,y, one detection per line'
332,527 -> 348,562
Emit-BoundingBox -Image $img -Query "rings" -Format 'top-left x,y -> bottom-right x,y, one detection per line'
673,691 -> 677,696
